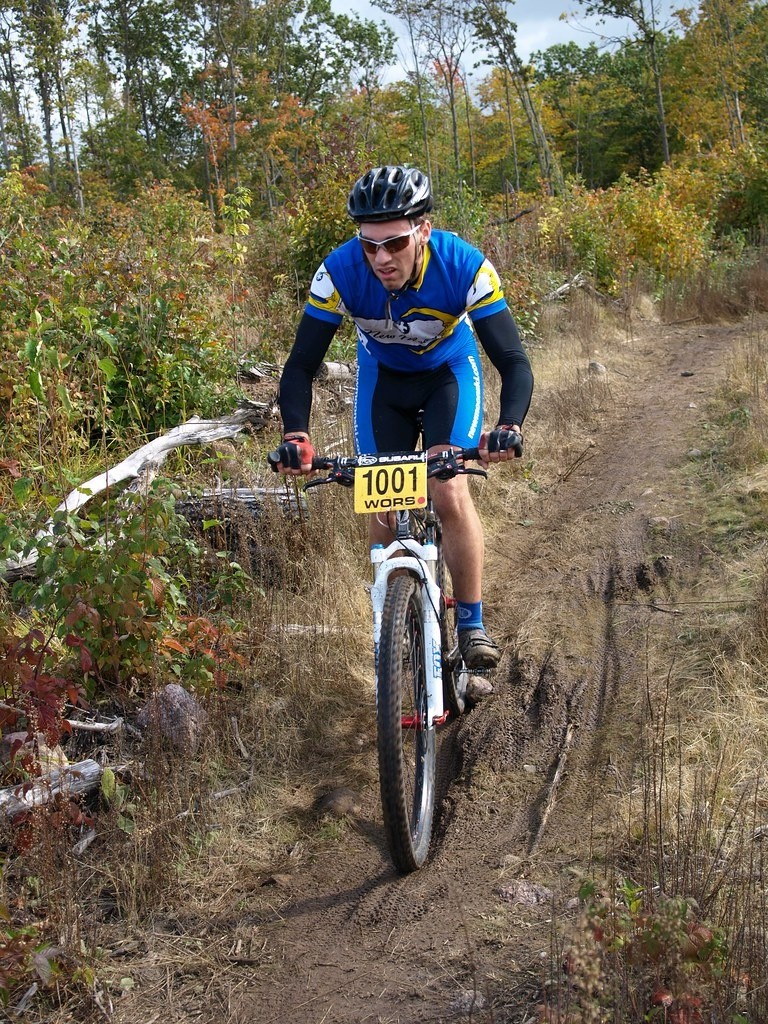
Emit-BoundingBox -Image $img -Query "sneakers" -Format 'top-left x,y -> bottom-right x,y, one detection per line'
459,626 -> 501,668
401,641 -> 412,666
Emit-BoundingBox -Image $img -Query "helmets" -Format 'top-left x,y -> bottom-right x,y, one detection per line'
345,166 -> 433,224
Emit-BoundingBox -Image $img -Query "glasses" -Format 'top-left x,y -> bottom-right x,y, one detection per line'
358,220 -> 424,254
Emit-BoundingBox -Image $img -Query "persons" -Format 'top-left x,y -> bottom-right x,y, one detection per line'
280,166 -> 534,670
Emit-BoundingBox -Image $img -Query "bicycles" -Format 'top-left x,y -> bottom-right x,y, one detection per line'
265,432 -> 526,876
